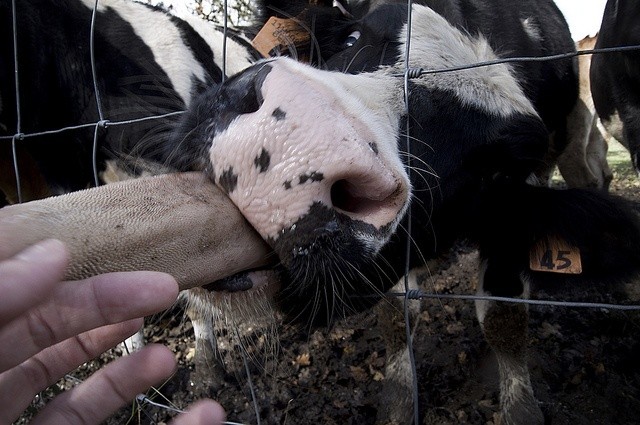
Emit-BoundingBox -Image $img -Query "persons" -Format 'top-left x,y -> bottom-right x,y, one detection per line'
1,238 -> 227,425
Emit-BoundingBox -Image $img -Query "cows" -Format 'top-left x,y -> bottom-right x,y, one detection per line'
165,1 -> 580,425
590,0 -> 640,180
0,0 -> 282,401
